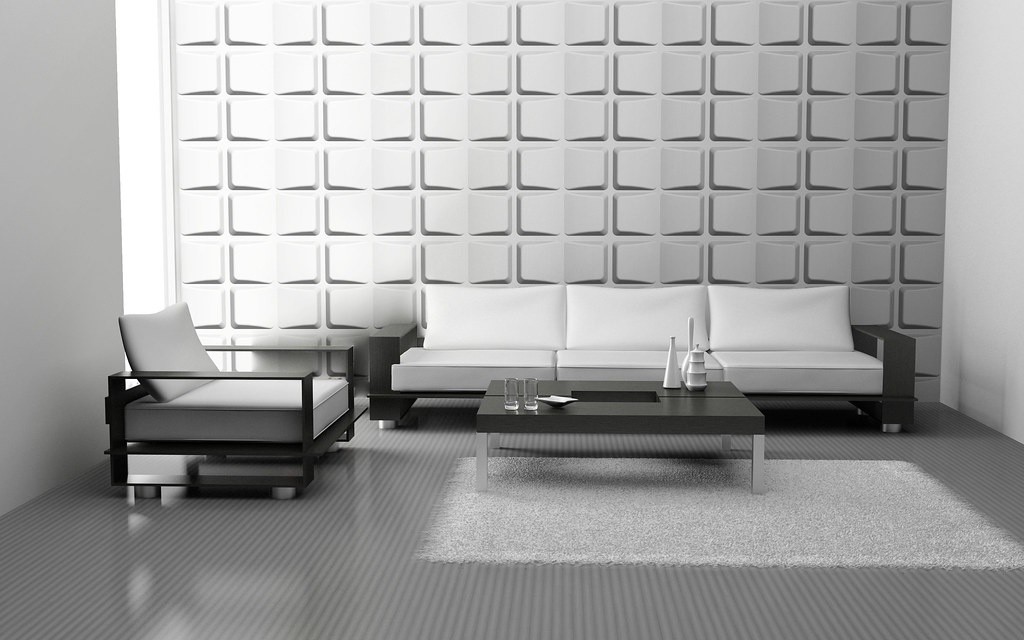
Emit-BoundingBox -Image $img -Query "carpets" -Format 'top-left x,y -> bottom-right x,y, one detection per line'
414,456 -> 1024,570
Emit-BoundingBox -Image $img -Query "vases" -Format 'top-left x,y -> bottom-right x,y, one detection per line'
662,336 -> 681,388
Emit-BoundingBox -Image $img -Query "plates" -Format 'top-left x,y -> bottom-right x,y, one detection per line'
535,395 -> 579,408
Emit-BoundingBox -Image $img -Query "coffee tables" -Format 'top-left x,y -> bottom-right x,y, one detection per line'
475,380 -> 764,495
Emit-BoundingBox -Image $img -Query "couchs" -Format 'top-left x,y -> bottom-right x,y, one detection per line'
366,285 -> 918,434
104,302 -> 356,500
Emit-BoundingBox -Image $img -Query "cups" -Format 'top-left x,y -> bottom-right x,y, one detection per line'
523,377 -> 538,411
505,377 -> 519,411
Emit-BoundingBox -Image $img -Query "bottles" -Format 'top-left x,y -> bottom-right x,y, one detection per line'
682,316 -> 694,385
686,343 -> 708,391
663,336 -> 682,389
